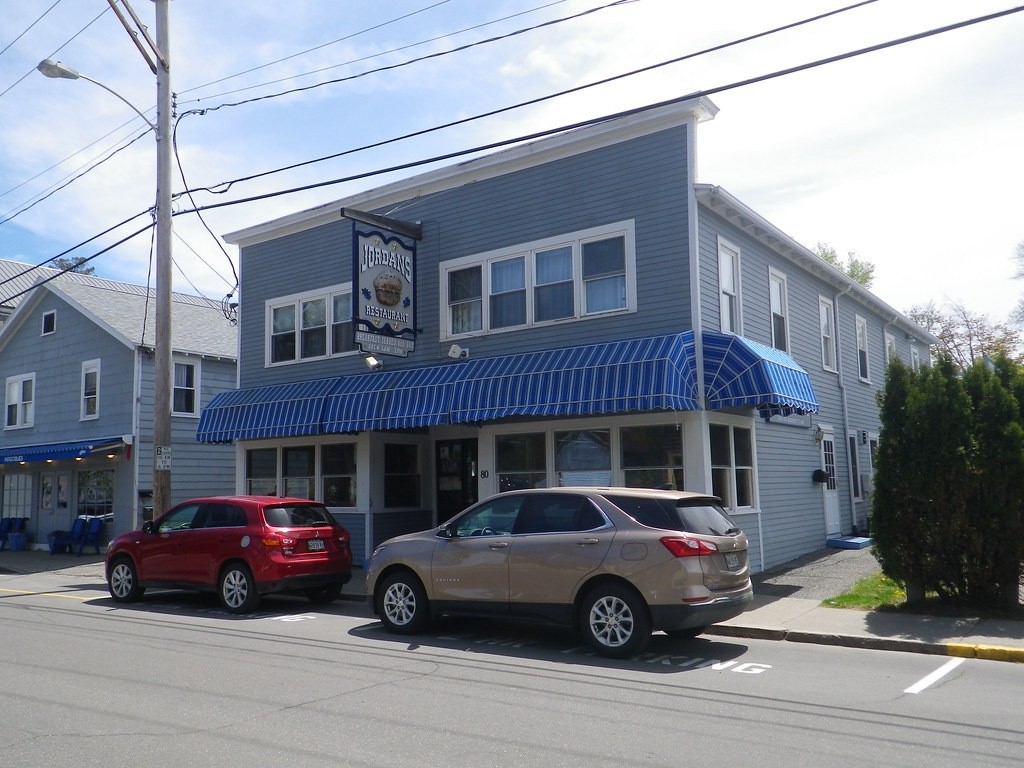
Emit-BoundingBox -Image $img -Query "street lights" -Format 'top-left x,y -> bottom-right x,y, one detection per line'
40,58 -> 174,523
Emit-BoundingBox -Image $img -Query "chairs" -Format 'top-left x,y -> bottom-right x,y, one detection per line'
46,519 -> 102,557
0,517 -> 30,552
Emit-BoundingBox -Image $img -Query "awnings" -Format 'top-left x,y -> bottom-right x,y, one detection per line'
0,439 -> 118,464
702,329 -> 818,419
196,330 -> 698,444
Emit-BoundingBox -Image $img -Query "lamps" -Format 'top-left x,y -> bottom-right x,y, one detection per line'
365,357 -> 383,370
905,334 -> 916,343
448,345 -> 470,359
814,425 -> 824,450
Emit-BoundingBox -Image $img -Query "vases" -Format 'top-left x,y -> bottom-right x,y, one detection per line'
8,532 -> 30,552
47,533 -> 70,555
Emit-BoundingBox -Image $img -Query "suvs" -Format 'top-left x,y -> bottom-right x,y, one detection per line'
105,494 -> 354,616
365,482 -> 755,660
